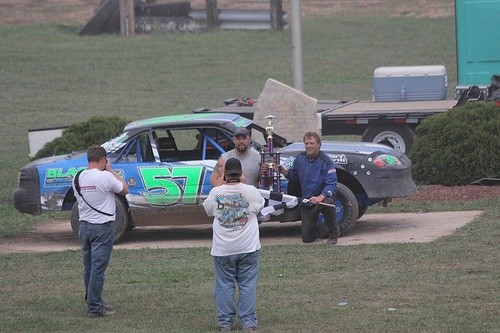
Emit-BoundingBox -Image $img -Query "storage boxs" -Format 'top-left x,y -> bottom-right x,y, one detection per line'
455,86 -> 489,100
373,65 -> 447,101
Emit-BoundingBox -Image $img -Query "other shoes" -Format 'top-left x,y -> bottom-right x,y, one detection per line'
326,233 -> 338,245
89,306 -> 116,317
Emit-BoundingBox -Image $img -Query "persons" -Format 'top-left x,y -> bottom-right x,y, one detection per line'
274,132 -> 340,245
72,143 -> 129,318
203,157 -> 265,331
486,75 -> 500,102
210,127 -> 269,225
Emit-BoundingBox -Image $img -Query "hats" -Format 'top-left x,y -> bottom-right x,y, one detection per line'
225,157 -> 242,176
234,126 -> 250,136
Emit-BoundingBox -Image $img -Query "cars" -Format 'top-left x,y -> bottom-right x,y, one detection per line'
12,112 -> 417,240
